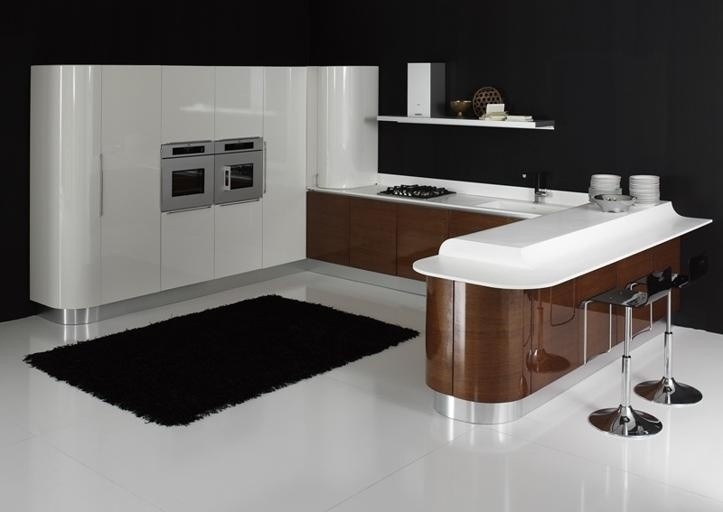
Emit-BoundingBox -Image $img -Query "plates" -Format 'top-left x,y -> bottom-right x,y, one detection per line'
629,174 -> 661,206
589,173 -> 622,190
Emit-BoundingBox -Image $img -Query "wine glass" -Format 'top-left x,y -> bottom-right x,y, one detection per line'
450,100 -> 472,118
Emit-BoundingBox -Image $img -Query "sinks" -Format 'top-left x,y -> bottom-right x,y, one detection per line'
476,199 -> 568,215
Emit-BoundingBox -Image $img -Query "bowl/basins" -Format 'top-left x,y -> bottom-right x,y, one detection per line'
589,193 -> 637,213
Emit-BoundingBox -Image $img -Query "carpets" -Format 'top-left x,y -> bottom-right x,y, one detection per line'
21,293 -> 422,429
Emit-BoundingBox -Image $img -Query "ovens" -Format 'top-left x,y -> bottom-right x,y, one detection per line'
161,137 -> 263,210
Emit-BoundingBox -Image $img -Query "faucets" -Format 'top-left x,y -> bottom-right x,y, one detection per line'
522,172 -> 546,203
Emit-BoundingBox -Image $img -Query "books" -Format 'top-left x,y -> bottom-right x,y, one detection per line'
506,115 -> 533,121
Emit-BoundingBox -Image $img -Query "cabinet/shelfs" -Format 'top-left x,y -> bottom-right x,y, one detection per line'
305,191 -> 350,266
395,203 -> 447,283
447,210 -> 509,237
214,65 -> 264,141
213,199 -> 262,280
102,65 -> 160,305
161,205 -> 213,293
307,65 -> 382,189
264,66 -> 306,269
161,65 -> 215,145
350,197 -> 396,276
25,65 -> 102,310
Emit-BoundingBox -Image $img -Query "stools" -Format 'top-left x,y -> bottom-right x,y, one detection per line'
630,250 -> 708,405
521,281 -> 576,375
581,266 -> 669,439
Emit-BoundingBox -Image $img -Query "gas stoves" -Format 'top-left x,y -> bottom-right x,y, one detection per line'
377,183 -> 455,201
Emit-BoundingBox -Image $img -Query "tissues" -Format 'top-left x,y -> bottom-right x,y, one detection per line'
479,104 -> 507,121
